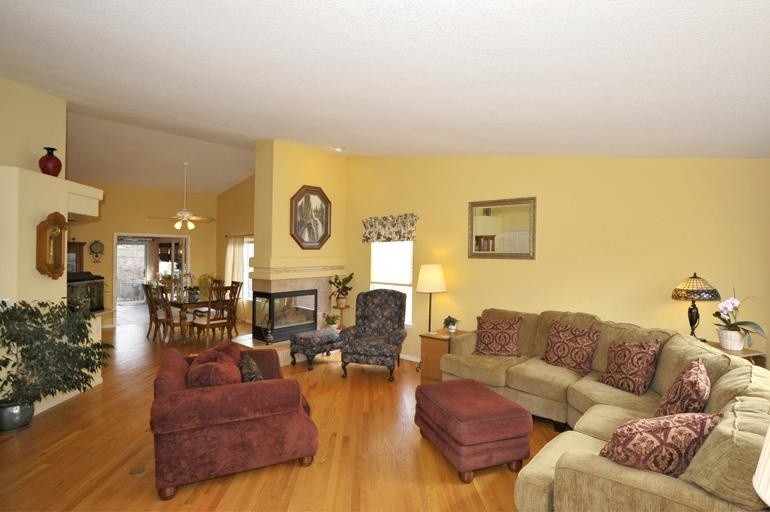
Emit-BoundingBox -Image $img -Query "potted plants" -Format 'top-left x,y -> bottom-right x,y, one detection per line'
0,296 -> 117,435
327,272 -> 355,307
442,315 -> 460,333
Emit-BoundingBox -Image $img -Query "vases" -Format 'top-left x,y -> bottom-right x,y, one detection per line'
715,328 -> 745,351
38,146 -> 63,177
327,324 -> 338,331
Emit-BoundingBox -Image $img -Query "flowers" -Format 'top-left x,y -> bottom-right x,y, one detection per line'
323,311 -> 341,324
710,285 -> 769,349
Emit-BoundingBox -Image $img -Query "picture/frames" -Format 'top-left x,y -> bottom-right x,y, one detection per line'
288,184 -> 333,251
467,197 -> 537,260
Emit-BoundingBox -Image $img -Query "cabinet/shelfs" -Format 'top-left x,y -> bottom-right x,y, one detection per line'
67,241 -> 88,273
419,328 -> 465,381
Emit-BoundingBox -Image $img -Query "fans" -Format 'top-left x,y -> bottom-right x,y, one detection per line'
144,160 -> 217,232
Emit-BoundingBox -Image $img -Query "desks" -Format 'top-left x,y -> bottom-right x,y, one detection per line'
702,341 -> 767,369
333,305 -> 350,333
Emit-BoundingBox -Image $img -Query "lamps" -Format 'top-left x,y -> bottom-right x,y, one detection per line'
416,263 -> 446,336
172,219 -> 196,233
671,272 -> 722,344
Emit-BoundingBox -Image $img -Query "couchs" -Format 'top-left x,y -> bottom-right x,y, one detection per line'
412,375 -> 535,486
148,339 -> 320,502
437,305 -> 770,512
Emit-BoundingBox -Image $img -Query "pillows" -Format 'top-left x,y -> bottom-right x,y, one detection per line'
651,354 -> 712,416
236,351 -> 263,384
598,337 -> 662,396
543,319 -> 601,377
597,410 -> 724,480
471,313 -> 523,359
184,340 -> 243,389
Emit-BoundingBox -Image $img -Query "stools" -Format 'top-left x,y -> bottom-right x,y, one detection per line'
289,329 -> 343,372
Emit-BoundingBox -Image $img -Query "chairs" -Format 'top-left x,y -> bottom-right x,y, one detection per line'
141,276 -> 244,347
337,288 -> 408,382
